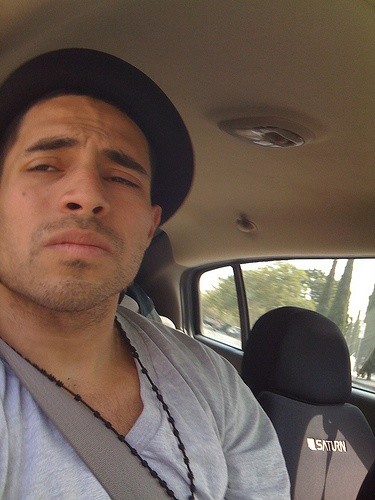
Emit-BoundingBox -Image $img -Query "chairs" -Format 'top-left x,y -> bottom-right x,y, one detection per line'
239,306 -> 375,500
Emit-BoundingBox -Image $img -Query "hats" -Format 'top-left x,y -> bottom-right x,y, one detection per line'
0,47 -> 195,227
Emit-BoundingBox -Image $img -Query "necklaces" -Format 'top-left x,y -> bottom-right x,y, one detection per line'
0,315 -> 197,500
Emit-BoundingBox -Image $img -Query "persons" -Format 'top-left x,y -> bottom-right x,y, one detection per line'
0,48 -> 292,500
